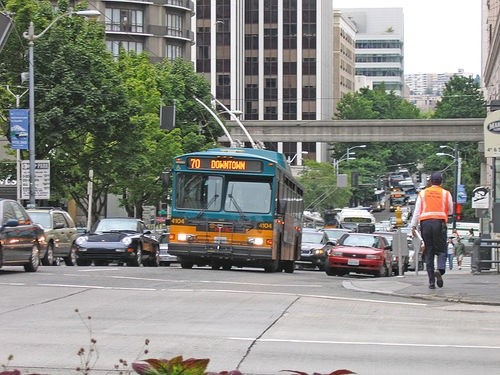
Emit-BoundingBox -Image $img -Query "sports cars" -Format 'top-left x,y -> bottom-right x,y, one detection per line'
71,217 -> 160,268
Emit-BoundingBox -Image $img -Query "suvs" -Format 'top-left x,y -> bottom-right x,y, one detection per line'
25,207 -> 78,267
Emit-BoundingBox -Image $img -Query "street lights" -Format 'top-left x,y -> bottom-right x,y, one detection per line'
333,152 -> 355,175
436,152 -> 462,185
440,146 -> 460,157
287,151 -> 309,167
346,145 -> 367,162
23,9 -> 101,208
198,110 -> 243,136
337,157 -> 356,174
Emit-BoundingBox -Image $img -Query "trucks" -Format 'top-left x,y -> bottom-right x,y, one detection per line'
372,232 -> 408,276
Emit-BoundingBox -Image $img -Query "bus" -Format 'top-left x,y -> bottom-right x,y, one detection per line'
363,190 -> 386,212
336,209 -> 375,234
166,147 -> 304,274
388,191 -> 409,212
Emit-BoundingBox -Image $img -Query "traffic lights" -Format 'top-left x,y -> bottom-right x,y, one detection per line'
453,215 -> 465,221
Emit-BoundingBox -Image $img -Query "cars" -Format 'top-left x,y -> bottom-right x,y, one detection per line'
294,231 -> 328,272
325,233 -> 392,277
0,197 -> 47,273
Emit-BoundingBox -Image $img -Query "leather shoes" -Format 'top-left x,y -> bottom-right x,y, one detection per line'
429,283 -> 436,288
434,270 -> 444,286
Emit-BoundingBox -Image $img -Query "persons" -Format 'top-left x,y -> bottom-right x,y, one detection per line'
410,171 -> 454,290
446,238 -> 455,270
453,236 -> 465,270
